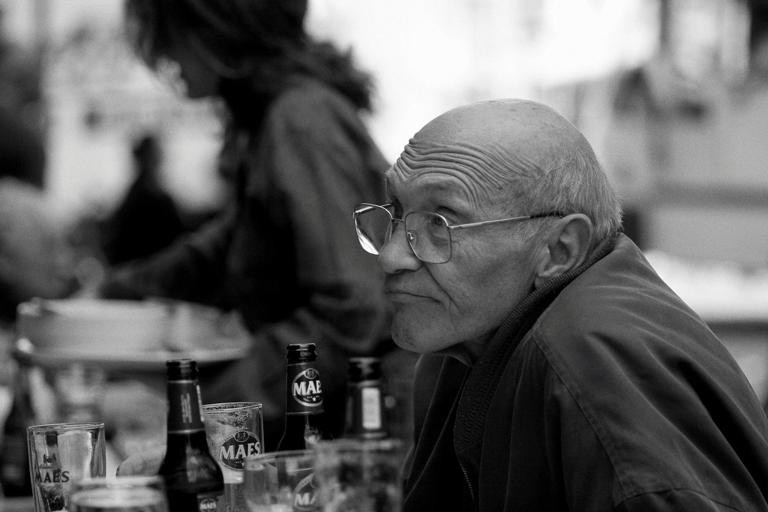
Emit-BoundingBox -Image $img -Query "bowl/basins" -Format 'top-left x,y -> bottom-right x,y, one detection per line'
13,298 -> 168,354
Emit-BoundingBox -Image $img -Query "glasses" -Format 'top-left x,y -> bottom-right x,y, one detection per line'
352,201 -> 567,264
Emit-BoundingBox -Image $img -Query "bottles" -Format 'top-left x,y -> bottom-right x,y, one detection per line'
144,357 -> 227,512
337,358 -> 401,436
272,342 -> 333,455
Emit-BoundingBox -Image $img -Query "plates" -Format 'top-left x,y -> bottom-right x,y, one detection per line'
13,330 -> 249,370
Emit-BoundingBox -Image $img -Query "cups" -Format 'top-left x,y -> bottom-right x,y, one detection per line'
200,401 -> 265,512
60,475 -> 170,512
241,450 -> 332,512
25,420 -> 108,512
308,438 -> 408,512
